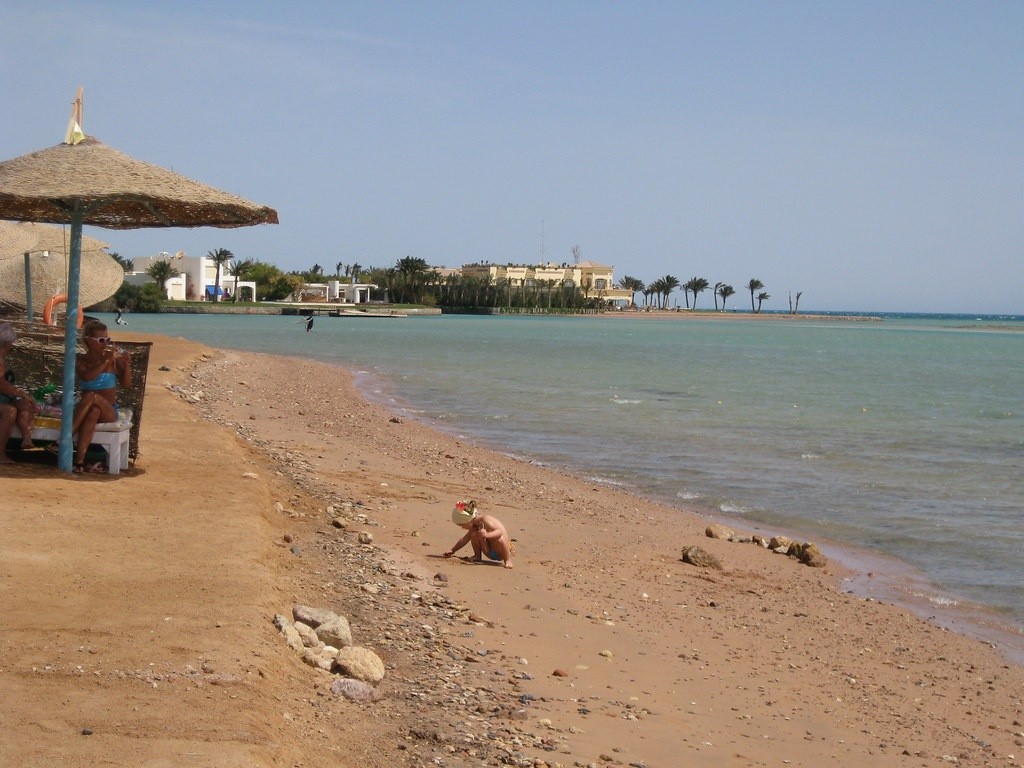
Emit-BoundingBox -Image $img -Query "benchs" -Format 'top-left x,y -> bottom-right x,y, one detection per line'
0,307 -> 154,476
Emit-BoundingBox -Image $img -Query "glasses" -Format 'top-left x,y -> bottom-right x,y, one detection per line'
85,336 -> 110,344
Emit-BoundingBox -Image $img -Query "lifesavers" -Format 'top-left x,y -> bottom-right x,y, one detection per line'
42,294 -> 84,328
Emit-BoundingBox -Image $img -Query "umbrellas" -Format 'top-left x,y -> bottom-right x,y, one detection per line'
0,134 -> 279,474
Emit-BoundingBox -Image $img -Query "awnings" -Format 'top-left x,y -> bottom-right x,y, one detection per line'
206,285 -> 224,295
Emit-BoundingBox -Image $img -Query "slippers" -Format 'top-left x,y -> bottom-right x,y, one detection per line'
73,462 -> 84,474
46,441 -> 59,454
22,445 -> 44,451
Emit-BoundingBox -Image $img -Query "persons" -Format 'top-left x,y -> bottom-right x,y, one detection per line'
0,338 -> 41,464
47,320 -> 131,473
304,314 -> 313,335
444,500 -> 514,569
115,308 -> 122,325
733,306 -> 736,313
